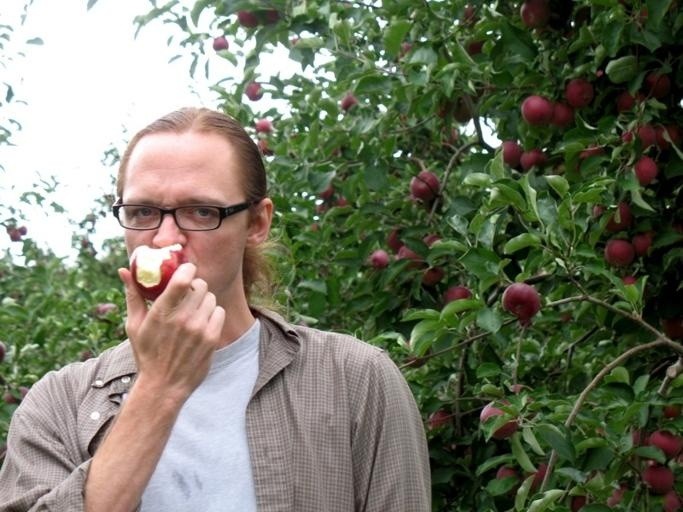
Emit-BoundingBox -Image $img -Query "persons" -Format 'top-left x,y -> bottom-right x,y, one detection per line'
0,106 -> 432,512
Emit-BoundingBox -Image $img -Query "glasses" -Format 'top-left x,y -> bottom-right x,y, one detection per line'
112,198 -> 260,231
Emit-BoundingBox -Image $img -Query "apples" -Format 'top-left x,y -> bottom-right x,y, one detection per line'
127,244 -> 197,302
0,0 -> 683,512
2,302 -> 128,408
6,224 -> 28,241
213,0 -> 682,512
